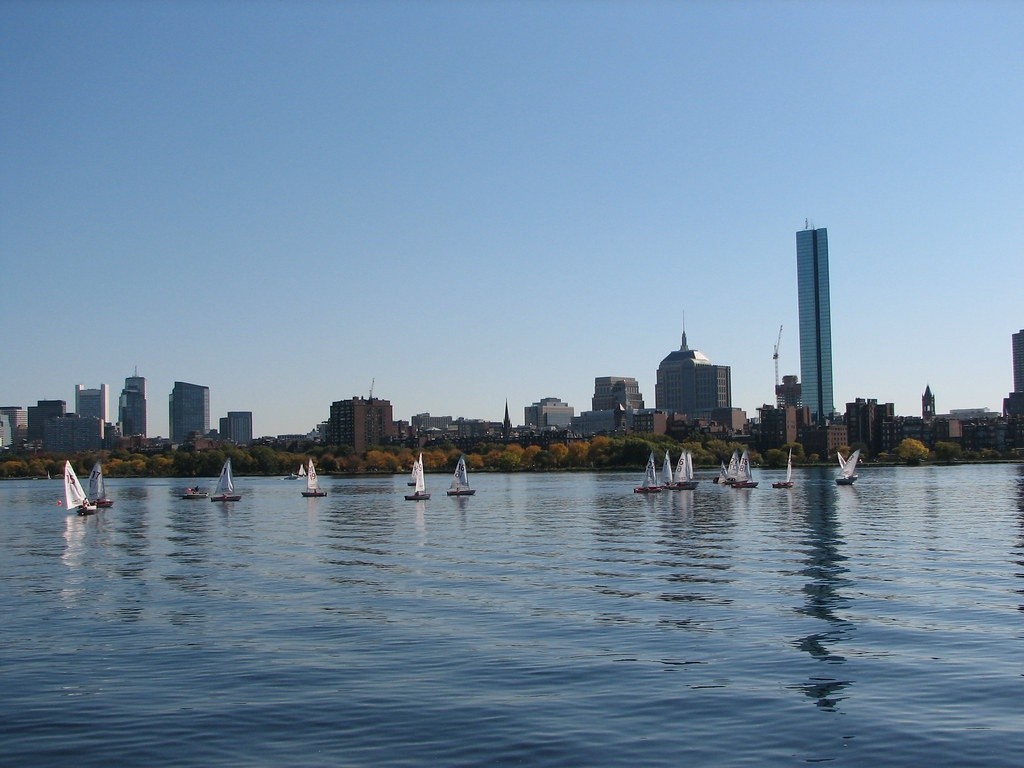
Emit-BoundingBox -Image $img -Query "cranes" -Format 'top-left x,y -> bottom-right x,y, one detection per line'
368,377 -> 376,398
772,326 -> 783,408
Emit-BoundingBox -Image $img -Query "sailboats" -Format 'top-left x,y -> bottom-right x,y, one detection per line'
210,458 -> 242,501
835,449 -> 860,486
301,458 -> 328,498
64,460 -> 96,515
634,450 -> 662,492
715,449 -> 739,483
772,448 -> 794,489
404,452 -> 431,500
84,460 -> 113,508
446,453 -> 477,496
732,449 -> 759,489
657,450 -> 700,490
284,464 -> 307,480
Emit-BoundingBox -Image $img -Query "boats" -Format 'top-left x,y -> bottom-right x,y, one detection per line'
182,492 -> 208,498
